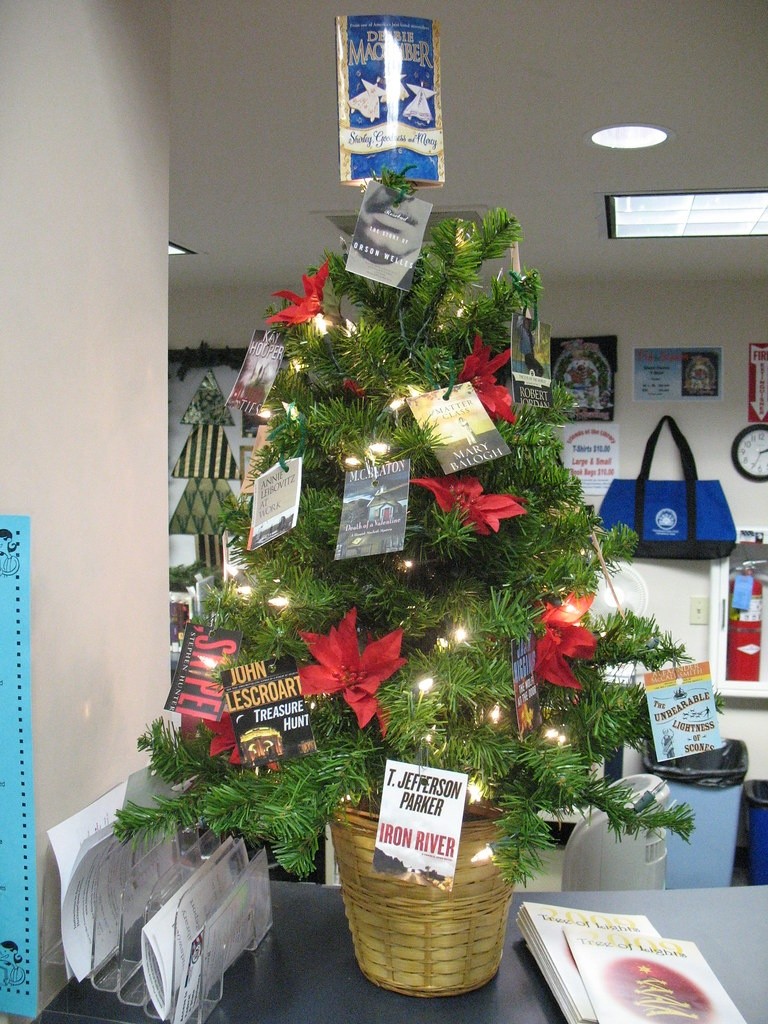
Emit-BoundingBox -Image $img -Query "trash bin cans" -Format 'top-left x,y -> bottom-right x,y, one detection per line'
641,737 -> 749,891
743,778 -> 768,886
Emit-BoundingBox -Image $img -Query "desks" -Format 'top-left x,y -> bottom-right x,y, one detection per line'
23,883 -> 768,1024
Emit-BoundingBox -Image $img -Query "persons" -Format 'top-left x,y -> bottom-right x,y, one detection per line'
515,316 -> 545,377
458,417 -> 478,445
345,180 -> 433,291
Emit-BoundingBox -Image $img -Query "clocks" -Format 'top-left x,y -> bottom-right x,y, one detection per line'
730,424 -> 768,482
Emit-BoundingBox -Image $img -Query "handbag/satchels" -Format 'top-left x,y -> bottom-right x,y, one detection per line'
588,415 -> 737,560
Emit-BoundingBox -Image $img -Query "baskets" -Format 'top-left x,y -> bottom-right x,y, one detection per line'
329,798 -> 520,999
